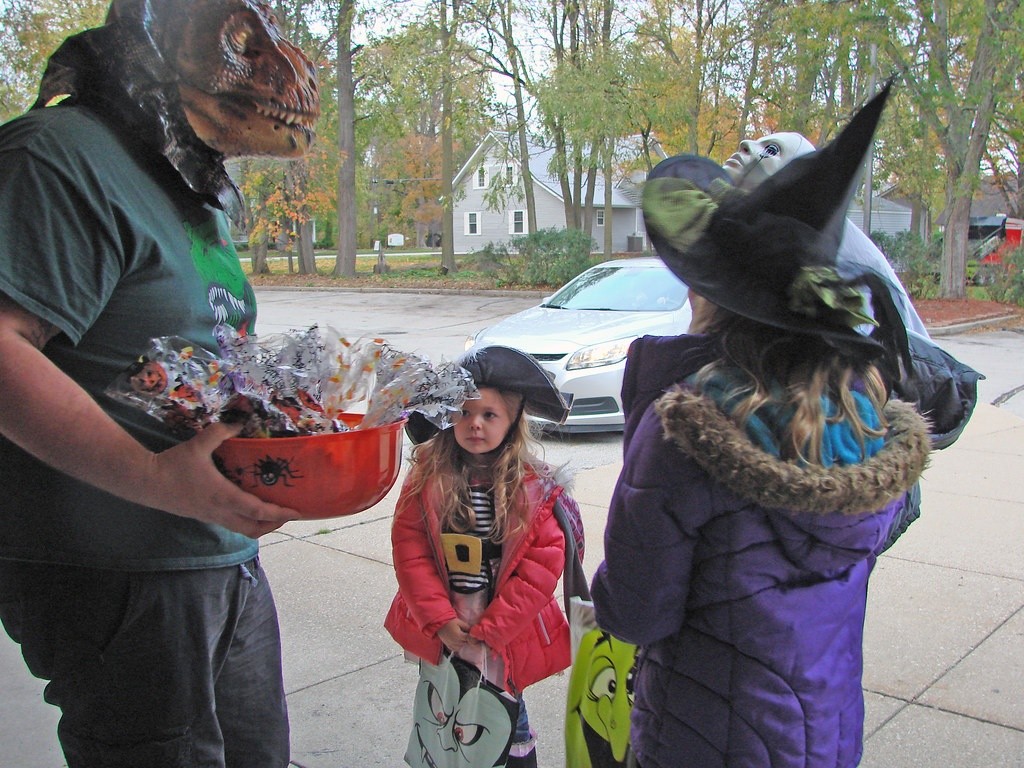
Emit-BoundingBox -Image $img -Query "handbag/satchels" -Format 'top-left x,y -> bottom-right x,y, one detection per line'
564,626 -> 645,768
404,640 -> 520,768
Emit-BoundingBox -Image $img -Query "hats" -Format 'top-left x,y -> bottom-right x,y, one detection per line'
629,71 -> 901,352
406,348 -> 569,446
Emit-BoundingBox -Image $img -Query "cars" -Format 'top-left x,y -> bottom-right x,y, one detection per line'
459,252 -> 692,435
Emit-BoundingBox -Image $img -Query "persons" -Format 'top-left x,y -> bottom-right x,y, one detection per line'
0,0 -> 321,768
382,348 -> 574,768
591,72 -> 937,768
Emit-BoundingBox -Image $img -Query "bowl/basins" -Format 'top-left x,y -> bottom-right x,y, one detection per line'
213,412 -> 409,521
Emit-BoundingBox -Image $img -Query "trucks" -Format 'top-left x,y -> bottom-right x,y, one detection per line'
925,213 -> 1024,285
